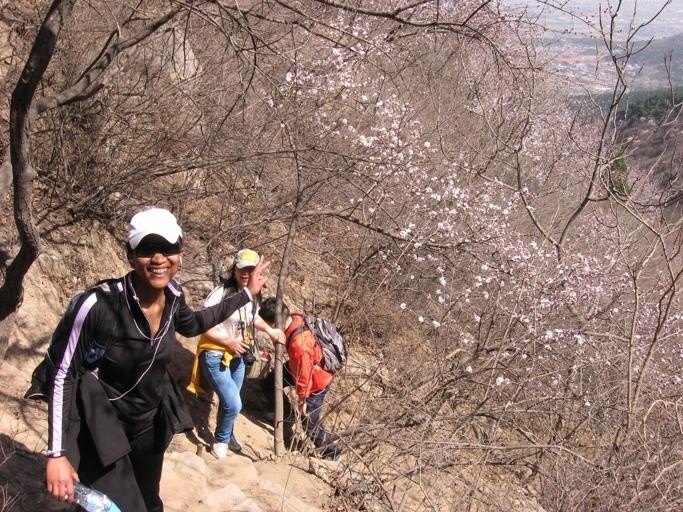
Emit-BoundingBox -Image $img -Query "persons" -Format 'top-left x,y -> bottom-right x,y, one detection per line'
44,207 -> 272,512
257,294 -> 344,459
184,250 -> 287,461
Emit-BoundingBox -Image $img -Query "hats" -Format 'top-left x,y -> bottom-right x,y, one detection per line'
234,249 -> 261,270
126,209 -> 184,250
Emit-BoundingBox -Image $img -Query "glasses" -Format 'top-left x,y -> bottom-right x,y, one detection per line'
136,238 -> 180,259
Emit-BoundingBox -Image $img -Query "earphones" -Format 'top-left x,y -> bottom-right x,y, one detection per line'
128,259 -> 134,264
179,256 -> 183,264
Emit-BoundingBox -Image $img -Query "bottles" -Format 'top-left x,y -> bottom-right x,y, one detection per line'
72,484 -> 121,512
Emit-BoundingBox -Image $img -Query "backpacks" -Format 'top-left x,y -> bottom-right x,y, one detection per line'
288,314 -> 348,374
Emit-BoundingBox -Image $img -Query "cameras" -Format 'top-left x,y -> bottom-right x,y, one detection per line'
242,342 -> 256,366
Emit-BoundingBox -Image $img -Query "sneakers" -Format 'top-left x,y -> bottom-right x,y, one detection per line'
210,435 -> 241,459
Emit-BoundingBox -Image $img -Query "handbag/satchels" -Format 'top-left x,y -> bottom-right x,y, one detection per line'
246,348 -> 271,380
